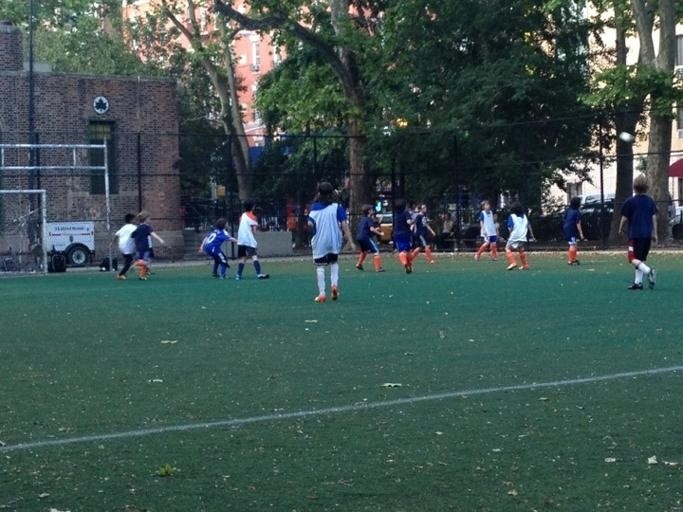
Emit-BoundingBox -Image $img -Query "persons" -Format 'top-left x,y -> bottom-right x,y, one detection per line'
235,199 -> 269,280
562,197 -> 588,265
473,200 -> 499,261
355,204 -> 385,273
504,202 -> 535,271
617,175 -> 658,289
198,218 -> 238,279
306,181 -> 356,303
112,213 -> 138,280
392,198 -> 458,274
130,211 -> 165,280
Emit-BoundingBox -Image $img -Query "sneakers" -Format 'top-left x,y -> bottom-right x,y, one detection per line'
116,270 -> 153,281
314,295 -> 325,303
628,283 -> 644,290
210,273 -> 270,280
331,286 -> 338,300
355,253 -> 530,274
647,267 -> 658,289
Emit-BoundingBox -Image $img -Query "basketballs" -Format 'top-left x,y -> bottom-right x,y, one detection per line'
620,131 -> 635,142
